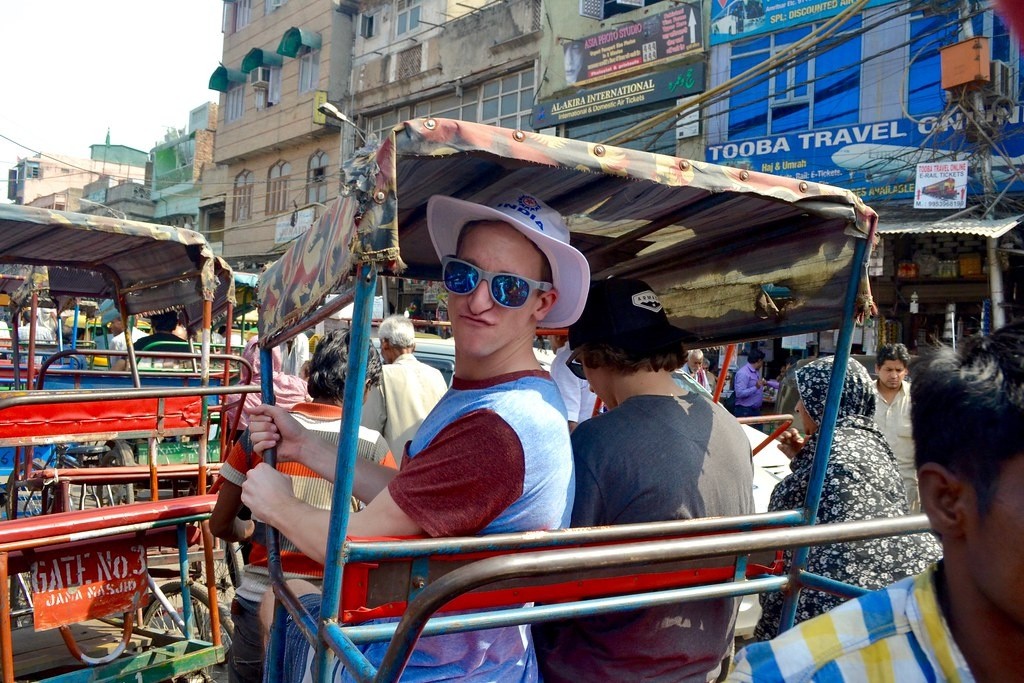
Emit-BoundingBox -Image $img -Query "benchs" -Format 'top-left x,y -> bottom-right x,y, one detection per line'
271,519 -> 788,683
0,370 -> 222,560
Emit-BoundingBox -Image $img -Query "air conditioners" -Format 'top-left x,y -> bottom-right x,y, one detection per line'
947,60 -> 1013,103
249,66 -> 270,88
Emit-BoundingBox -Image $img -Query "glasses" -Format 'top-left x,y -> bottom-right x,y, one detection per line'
441,254 -> 552,310
565,343 -> 587,380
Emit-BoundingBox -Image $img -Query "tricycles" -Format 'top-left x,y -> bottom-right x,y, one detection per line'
0,117 -> 941,683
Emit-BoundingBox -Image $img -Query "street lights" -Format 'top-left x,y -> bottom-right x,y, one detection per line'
77,198 -> 127,220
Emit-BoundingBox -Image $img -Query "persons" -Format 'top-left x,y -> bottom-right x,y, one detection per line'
565,36 -> 594,84
0,189 -> 1024,683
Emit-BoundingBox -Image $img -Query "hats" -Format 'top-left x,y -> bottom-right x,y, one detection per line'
427,188 -> 590,328
569,279 -> 702,350
